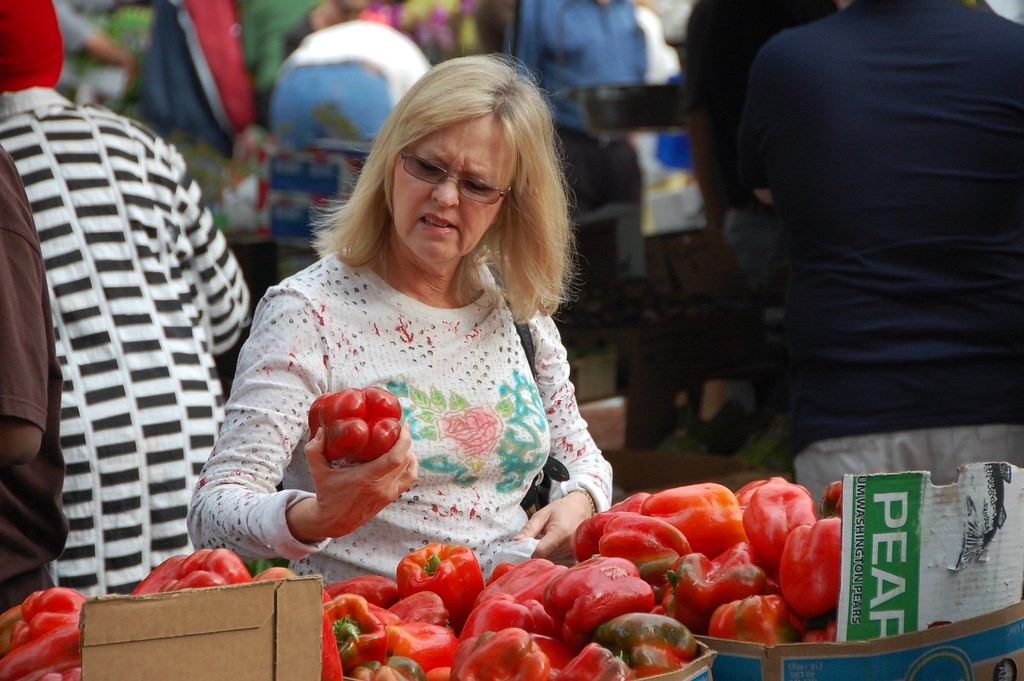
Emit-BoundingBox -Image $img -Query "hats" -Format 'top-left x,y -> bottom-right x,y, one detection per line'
0,0 -> 63,94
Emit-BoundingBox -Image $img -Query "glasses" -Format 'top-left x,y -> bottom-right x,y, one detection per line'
401,151 -> 512,205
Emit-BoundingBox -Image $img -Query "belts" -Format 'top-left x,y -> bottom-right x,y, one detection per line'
558,129 -> 626,151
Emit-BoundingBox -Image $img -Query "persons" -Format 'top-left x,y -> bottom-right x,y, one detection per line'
238,0 -> 369,100
52,0 -> 130,68
0,144 -> 70,613
487,0 -> 681,328
722,0 -> 1024,514
0,0 -> 250,600
185,54 -> 614,587
677,0 -> 839,232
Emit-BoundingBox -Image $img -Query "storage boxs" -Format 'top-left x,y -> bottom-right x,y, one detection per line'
695,598 -> 1024,681
270,135 -> 365,237
602,439 -> 793,496
637,642 -> 719,681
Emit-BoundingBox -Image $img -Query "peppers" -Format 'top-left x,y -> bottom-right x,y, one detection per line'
307,385 -> 402,468
572,476 -> 842,642
0,540 -> 699,681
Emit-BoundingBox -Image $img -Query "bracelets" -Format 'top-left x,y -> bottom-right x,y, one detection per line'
573,489 -> 597,517
